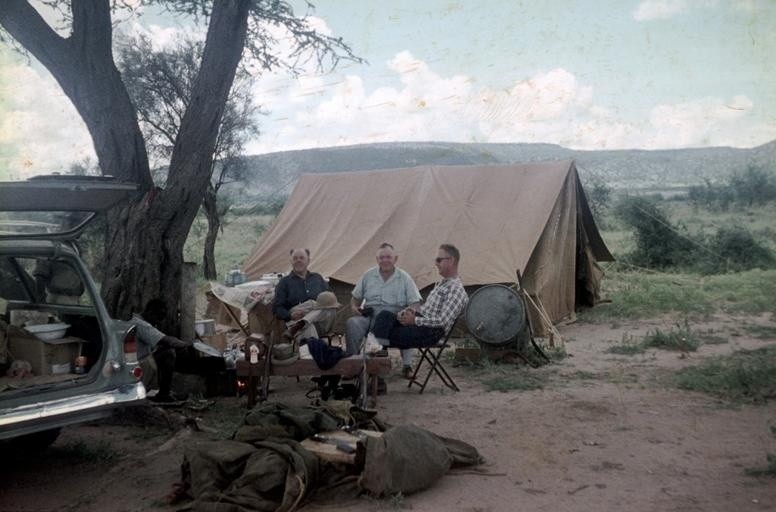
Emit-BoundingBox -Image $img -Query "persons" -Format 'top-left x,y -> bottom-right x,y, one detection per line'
130,295 -> 192,384
147,310 -> 202,403
345,243 -> 423,378
271,248 -> 336,348
357,244 -> 469,395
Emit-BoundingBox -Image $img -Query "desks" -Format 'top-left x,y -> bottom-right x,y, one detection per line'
235,356 -> 392,409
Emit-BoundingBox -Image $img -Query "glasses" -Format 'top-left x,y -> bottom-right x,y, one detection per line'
436,256 -> 450,261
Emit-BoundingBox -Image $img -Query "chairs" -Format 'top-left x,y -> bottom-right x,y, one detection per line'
408,306 -> 463,393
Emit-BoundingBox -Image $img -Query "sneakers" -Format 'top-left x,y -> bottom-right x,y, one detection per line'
361,382 -> 386,395
401,366 -> 415,379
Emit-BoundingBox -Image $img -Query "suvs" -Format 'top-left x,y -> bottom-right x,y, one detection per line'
1,171 -> 154,447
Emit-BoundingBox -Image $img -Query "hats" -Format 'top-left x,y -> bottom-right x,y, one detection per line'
272,343 -> 298,366
312,290 -> 343,309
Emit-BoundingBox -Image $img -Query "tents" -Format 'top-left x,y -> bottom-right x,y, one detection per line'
241,158 -> 616,337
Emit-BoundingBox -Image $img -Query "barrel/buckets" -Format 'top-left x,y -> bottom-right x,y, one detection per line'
224,264 -> 245,287
464,284 -> 529,348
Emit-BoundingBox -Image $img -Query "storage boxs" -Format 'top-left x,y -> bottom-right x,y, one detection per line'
9,331 -> 87,376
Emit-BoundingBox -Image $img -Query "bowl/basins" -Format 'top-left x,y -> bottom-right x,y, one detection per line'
23,323 -> 72,342
